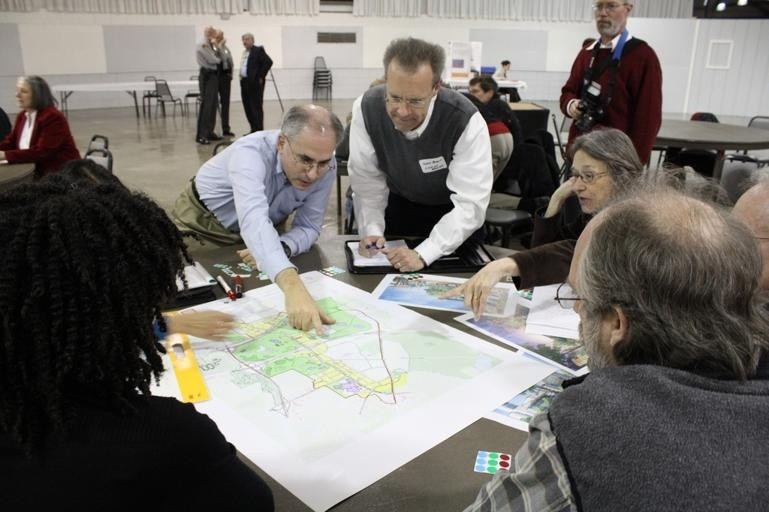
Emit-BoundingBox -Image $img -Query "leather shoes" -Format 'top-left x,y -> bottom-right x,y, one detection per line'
208,135 -> 223,141
195,136 -> 210,145
223,132 -> 235,137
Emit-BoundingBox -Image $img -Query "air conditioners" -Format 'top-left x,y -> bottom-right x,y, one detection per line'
318,0 -> 354,14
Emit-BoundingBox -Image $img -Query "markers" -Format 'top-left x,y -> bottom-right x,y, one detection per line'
235,276 -> 243,298
216,275 -> 236,301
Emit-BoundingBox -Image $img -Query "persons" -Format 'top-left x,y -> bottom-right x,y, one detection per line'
0,72 -> 82,168
456,164 -> 768,512
165,101 -> 348,335
718,162 -> 768,318
0,157 -> 278,512
348,35 -> 494,276
435,124 -> 645,322
451,90 -> 549,219
490,59 -> 529,106
191,24 -> 224,146
237,32 -> 274,137
211,29 -> 235,137
0,105 -> 14,143
558,0 -> 663,230
466,75 -> 529,193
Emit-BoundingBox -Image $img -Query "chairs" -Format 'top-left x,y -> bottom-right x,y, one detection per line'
313,55 -> 332,101
184,75 -> 201,118
86,134 -> 108,157
83,149 -> 114,174
142,76 -> 166,119
154,79 -> 184,120
729,115 -> 769,168
552,116 -> 570,180
689,111 -> 720,124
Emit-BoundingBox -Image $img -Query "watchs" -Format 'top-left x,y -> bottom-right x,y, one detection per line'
415,248 -> 430,272
279,241 -> 293,258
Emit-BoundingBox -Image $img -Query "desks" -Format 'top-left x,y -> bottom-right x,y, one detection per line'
49,79 -> 200,119
653,118 -> 769,203
1,162 -> 35,196
444,76 -> 524,103
155,229 -> 587,512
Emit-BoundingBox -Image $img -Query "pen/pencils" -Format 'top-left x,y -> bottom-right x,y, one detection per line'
365,241 -> 385,250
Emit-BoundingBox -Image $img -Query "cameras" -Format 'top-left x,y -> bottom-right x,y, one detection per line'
574,77 -> 607,132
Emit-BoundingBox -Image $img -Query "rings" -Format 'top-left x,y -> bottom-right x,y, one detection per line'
397,259 -> 404,269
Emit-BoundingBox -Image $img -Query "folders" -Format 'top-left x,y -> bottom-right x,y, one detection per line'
344,237 -> 496,274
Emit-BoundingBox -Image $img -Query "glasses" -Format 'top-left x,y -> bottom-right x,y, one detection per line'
566,167 -> 609,185
241,38 -> 251,41
591,1 -> 627,10
281,132 -> 337,172
553,281 -> 588,310
383,77 -> 435,108
